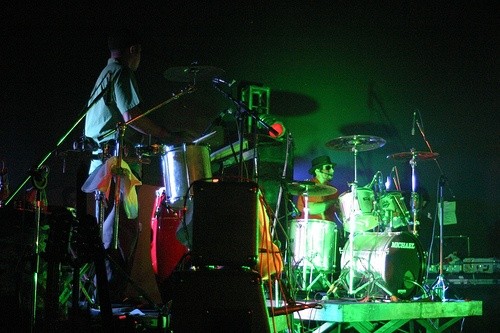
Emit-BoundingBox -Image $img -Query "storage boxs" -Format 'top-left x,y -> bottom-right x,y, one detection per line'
425,259 -> 496,284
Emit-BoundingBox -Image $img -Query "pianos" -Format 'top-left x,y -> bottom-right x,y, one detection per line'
207,133 -> 294,167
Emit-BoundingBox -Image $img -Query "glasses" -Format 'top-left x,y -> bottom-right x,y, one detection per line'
321,166 -> 334,171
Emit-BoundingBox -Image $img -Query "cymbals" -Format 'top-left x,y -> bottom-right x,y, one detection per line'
325,134 -> 387,152
164,64 -> 225,82
387,151 -> 440,159
285,181 -> 338,197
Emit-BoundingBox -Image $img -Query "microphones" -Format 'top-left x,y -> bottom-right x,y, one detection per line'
269,303 -> 317,317
412,111 -> 416,136
269,123 -> 283,137
105,70 -> 114,105
290,200 -> 301,217
369,171 -> 379,189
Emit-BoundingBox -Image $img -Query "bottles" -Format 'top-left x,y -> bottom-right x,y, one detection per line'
431,276 -> 446,302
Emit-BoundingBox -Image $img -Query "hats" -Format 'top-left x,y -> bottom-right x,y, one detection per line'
309,156 -> 336,174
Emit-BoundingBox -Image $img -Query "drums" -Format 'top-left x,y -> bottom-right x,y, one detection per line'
160,141 -> 215,210
339,187 -> 378,232
375,191 -> 414,229
339,232 -> 424,300
284,217 -> 338,273
149,186 -> 191,295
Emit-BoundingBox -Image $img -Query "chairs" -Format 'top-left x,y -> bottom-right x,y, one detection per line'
152,187 -> 191,276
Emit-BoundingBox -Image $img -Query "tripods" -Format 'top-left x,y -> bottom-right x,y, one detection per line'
288,120 -> 460,304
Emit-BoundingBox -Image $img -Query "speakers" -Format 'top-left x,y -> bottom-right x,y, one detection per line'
170,181 -> 275,333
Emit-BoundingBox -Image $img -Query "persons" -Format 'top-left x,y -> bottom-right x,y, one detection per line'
296,156 -> 341,221
398,186 -> 430,232
84,21 -> 183,306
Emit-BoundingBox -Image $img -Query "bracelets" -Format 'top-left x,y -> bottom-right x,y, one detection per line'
166,133 -> 176,142
